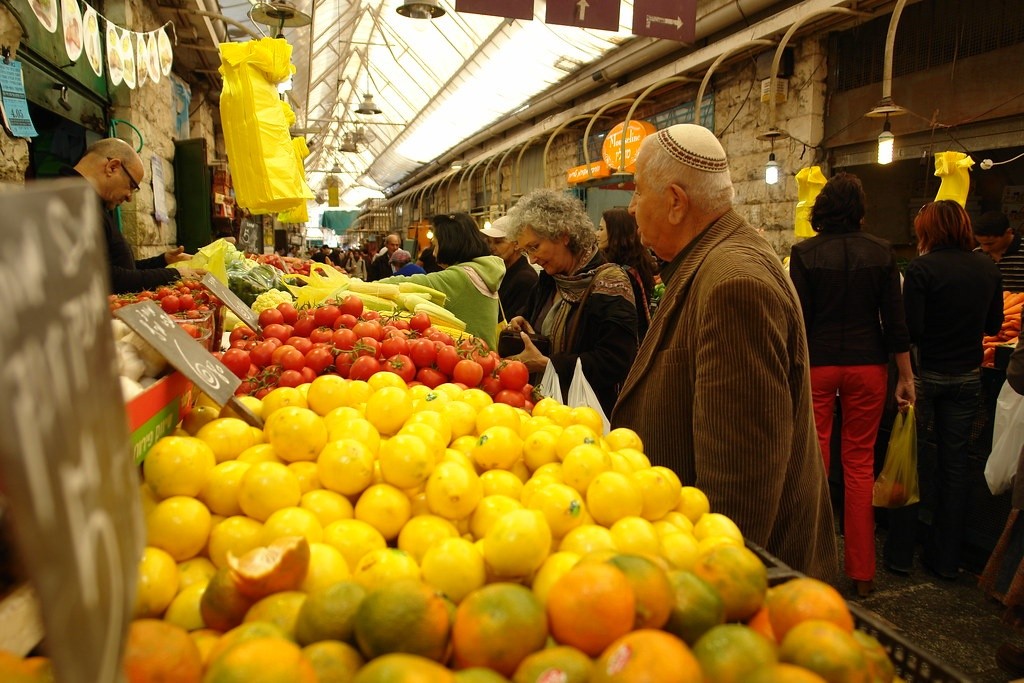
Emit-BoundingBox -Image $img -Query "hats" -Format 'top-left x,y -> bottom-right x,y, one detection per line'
389,249 -> 411,264
479,215 -> 511,238
656,123 -> 729,172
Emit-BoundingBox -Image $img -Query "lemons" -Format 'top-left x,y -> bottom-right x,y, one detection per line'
130,372 -> 745,619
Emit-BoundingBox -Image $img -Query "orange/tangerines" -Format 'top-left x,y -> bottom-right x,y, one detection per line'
0,534 -> 910,683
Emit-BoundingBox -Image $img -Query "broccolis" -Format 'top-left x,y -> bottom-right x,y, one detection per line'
227,262 -> 292,313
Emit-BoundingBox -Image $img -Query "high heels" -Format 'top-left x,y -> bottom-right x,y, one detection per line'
854,579 -> 872,597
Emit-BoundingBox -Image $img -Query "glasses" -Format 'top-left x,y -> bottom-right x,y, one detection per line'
520,246 -> 537,258
106,157 -> 140,192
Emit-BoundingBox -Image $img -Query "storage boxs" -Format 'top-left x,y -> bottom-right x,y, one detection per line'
124,370 -> 192,466
746,537 -> 975,683
211,161 -> 236,217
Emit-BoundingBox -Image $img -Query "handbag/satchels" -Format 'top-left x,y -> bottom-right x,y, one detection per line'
537,355 -> 611,437
496,294 -> 508,348
872,405 -> 920,507
984,379 -> 1024,496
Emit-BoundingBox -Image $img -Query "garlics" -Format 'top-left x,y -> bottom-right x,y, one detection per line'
113,320 -> 168,402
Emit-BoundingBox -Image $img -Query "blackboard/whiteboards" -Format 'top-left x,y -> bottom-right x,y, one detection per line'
200,272 -> 260,333
111,298 -> 242,407
239,221 -> 259,248
0,180 -> 146,683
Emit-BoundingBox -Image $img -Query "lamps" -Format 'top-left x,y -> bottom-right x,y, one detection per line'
338,104 -> 356,152
396,0 -> 446,19
354,44 -> 382,115
330,148 -> 341,173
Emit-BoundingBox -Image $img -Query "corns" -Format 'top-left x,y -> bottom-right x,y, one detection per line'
339,279 -> 474,340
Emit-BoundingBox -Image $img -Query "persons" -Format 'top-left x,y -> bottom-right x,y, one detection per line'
49,138 -> 207,295
505,191 -> 658,423
275,232 -> 379,283
791,173 -> 916,597
979,302 -> 1024,628
881,201 -> 1005,581
972,207 -> 1024,294
373,212 -> 544,358
609,124 -> 837,583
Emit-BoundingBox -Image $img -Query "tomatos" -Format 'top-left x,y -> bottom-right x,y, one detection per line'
106,276 -> 223,354
245,253 -> 328,279
207,295 -> 550,416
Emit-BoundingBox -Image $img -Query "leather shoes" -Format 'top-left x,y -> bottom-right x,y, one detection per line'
995,639 -> 1024,677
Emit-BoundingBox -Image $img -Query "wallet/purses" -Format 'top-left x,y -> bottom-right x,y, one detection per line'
497,330 -> 552,359
